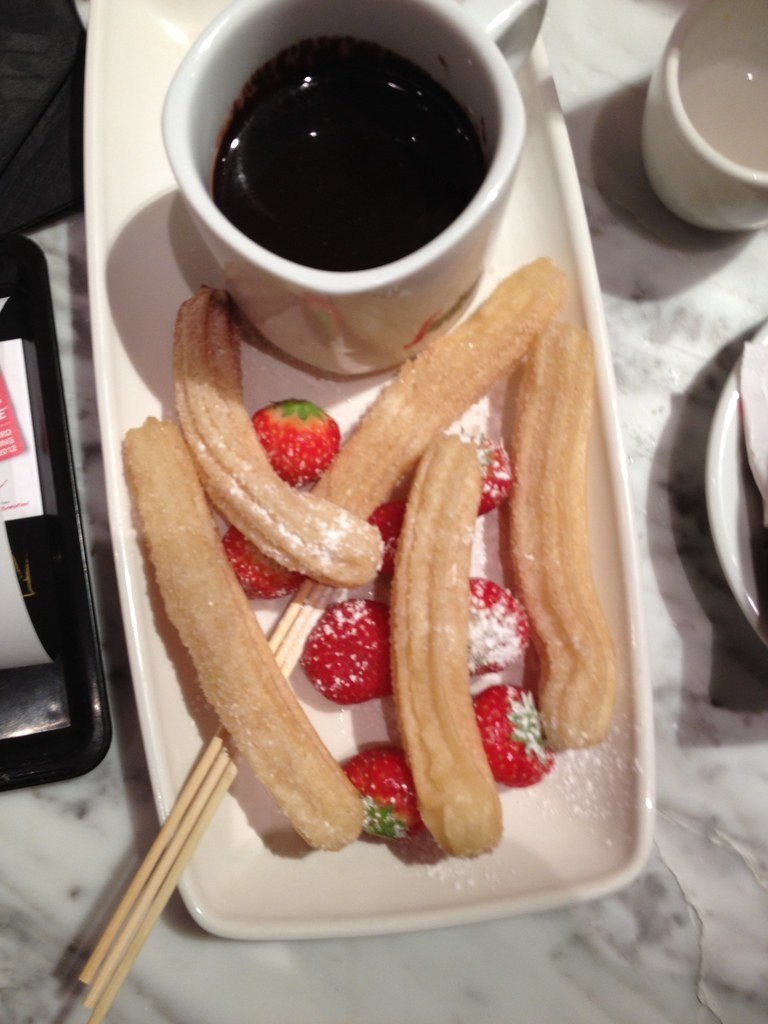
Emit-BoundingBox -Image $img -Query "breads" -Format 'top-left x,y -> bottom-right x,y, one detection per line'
513,318 -> 618,753
123,422 -> 368,850
172,289 -> 383,587
390,431 -> 507,858
308,255 -> 571,519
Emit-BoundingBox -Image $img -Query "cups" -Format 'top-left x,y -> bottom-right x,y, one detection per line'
643,0 -> 768,232
164,0 -> 529,385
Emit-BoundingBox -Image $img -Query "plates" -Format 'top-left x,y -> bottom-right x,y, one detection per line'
0,229 -> 111,793
78,0 -> 660,949
707,315 -> 768,645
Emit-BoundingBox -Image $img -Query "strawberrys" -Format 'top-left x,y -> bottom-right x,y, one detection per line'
215,400 -> 553,838
